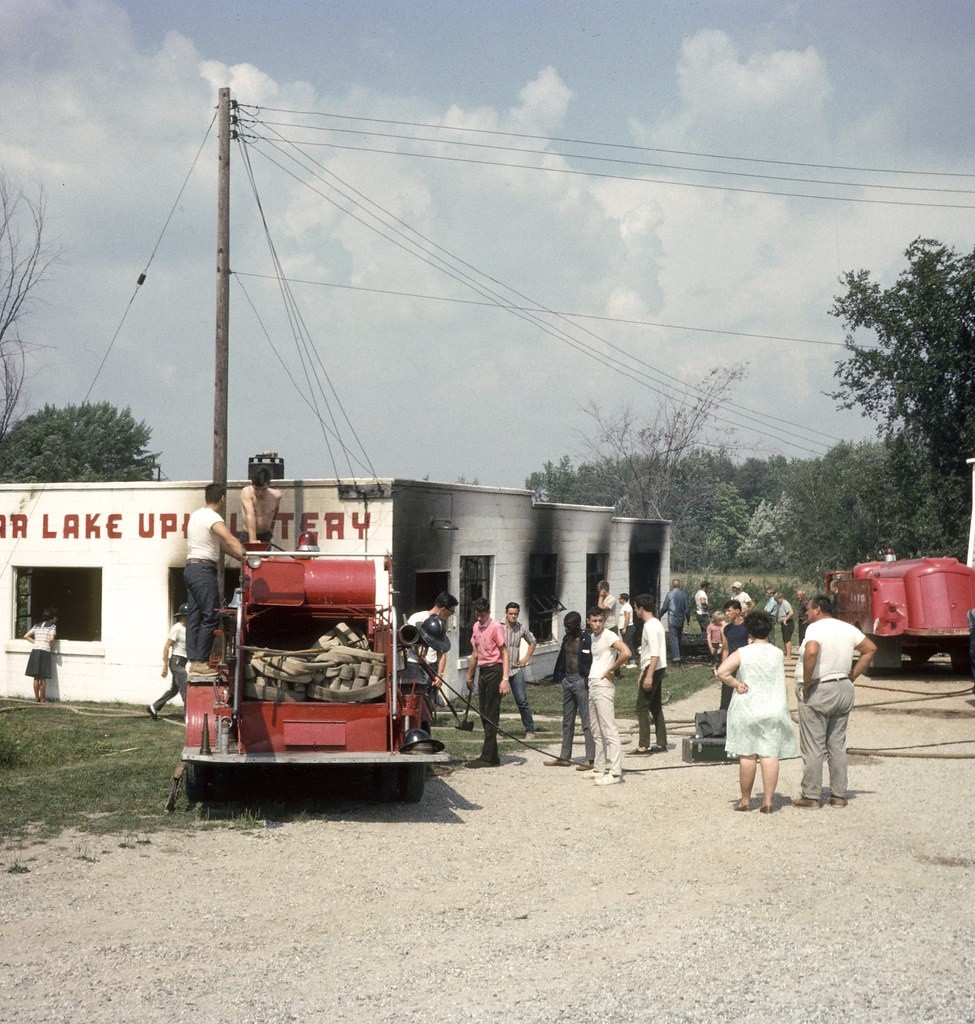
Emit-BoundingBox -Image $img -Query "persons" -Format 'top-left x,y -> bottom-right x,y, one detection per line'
763,579 -> 839,661
500,602 -> 537,739
625,595 -> 668,757
24,606 -> 58,702
597,580 -> 618,636
619,594 -> 638,669
715,612 -> 796,813
242,467 -> 281,544
147,603 -> 189,721
695,581 -> 754,710
658,579 -> 690,667
793,595 -> 877,808
544,611 -> 595,771
583,606 -> 632,784
183,483 -> 245,677
400,593 -> 458,691
466,598 -> 512,768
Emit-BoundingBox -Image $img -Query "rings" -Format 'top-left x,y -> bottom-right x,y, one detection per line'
439,685 -> 440,686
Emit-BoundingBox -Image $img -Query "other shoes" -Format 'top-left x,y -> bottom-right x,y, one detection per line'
825,795 -> 845,807
576,763 -> 592,770
544,759 -> 571,766
146,707 -> 157,720
734,804 -> 752,811
760,806 -> 772,813
793,797 -> 819,809
649,746 -> 667,754
465,757 -> 499,768
625,748 -> 649,756
526,732 -> 535,740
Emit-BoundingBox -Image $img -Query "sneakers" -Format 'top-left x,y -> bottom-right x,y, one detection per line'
188,661 -> 219,676
595,774 -> 620,785
583,768 -> 603,779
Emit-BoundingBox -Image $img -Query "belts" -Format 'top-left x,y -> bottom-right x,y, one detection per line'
188,558 -> 208,563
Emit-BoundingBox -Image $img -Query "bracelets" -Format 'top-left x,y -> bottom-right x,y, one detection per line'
503,678 -> 509,680
441,673 -> 443,675
608,670 -> 614,674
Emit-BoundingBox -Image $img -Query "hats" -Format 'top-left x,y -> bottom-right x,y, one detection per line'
731,581 -> 742,590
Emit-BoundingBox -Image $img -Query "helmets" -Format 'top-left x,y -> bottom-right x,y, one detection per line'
415,616 -> 452,653
172,603 -> 189,617
399,729 -> 445,752
